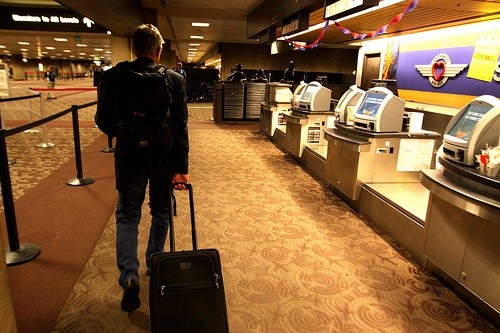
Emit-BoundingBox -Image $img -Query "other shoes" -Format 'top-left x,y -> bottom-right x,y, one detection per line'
120,278 -> 141,312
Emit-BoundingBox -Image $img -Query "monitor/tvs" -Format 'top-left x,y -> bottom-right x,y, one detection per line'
293,81 -> 331,111
443,95 -> 500,166
335,87 -> 366,123
352,87 -> 405,133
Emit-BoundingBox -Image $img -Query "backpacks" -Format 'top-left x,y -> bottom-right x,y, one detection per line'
115,60 -> 174,164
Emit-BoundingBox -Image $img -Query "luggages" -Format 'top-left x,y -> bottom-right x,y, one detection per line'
148,181 -> 229,333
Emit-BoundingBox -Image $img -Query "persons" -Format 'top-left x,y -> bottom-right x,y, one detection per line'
95,24 -> 190,310
284,61 -> 295,83
46,65 -> 57,99
227,64 -> 248,82
251,68 -> 268,82
173,62 -> 187,84
